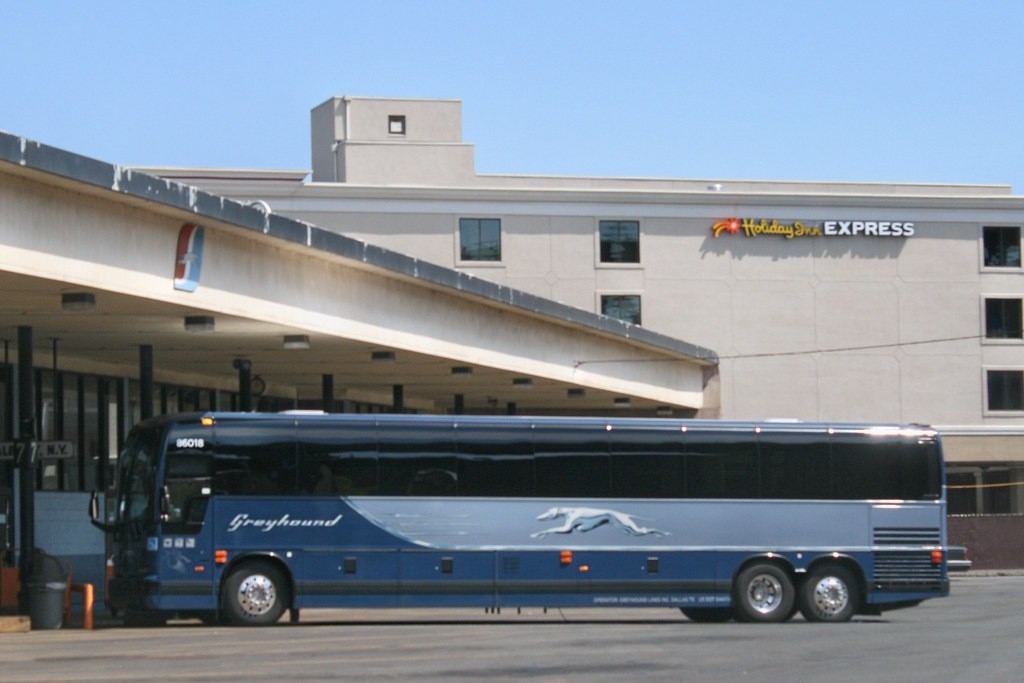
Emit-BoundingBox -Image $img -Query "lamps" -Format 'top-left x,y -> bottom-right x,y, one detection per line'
370,350 -> 675,417
283,335 -> 310,350
61,293 -> 96,311
184,315 -> 215,333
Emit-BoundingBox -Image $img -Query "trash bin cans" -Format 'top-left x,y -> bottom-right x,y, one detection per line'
24,583 -> 68,631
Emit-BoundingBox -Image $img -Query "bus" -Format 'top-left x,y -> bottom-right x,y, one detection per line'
89,409 -> 952,626
